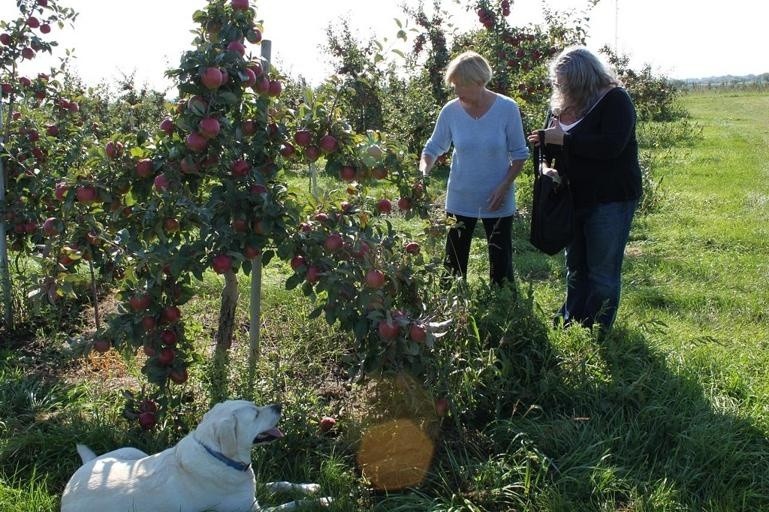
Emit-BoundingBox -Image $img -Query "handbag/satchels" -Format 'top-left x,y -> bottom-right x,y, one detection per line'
531,129 -> 575,256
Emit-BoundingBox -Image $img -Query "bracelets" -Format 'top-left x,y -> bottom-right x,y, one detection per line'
500,183 -> 509,191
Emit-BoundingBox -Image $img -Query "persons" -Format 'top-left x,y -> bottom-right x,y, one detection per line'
527,49 -> 644,334
419,50 -> 530,299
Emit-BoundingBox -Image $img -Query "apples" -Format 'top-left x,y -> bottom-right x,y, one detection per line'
0,0 -> 146,306
275,129 -> 451,426
124,1 -> 282,430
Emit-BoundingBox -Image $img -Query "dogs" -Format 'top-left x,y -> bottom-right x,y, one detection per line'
59,399 -> 336,511
